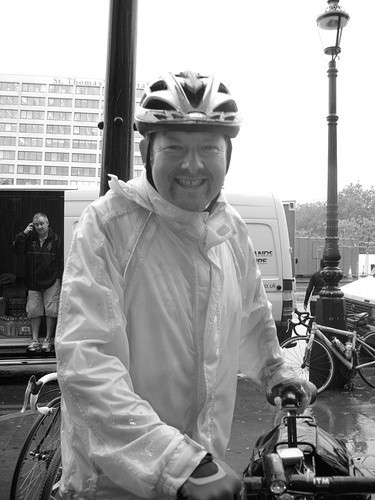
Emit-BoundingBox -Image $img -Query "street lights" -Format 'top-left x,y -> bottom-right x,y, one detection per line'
308,1 -> 352,393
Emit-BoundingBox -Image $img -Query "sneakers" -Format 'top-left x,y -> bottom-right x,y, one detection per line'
41,338 -> 53,353
26,340 -> 42,353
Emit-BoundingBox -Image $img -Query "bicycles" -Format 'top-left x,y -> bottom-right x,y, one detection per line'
10,373 -> 62,500
239,382 -> 374,500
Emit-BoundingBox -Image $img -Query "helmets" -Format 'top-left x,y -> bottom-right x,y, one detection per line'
135,71 -> 242,138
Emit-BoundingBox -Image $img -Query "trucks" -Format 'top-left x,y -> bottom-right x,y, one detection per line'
0,185 -> 297,375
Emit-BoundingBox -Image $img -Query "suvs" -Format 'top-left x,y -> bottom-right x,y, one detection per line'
339,266 -> 375,358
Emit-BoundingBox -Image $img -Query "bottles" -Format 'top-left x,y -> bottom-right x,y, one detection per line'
0,312 -> 32,337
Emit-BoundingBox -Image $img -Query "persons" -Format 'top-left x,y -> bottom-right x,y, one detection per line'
304,272 -> 319,310
13,213 -> 62,352
56,70 -> 316,500
